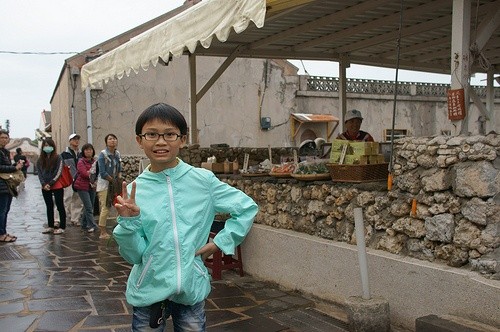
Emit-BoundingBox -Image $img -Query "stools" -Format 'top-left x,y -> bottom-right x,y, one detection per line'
204,219 -> 243,280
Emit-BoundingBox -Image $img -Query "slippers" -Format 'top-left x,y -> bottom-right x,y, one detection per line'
7,234 -> 17,239
0,236 -> 16,242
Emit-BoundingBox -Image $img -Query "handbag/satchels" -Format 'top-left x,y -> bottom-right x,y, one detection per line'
52,155 -> 73,189
1,169 -> 25,189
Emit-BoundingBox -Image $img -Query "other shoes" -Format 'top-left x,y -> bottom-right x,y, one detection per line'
88,228 -> 94,232
99,233 -> 111,239
53,228 -> 65,234
42,228 -> 54,233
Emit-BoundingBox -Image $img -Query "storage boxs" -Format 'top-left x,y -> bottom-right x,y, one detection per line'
327,162 -> 388,183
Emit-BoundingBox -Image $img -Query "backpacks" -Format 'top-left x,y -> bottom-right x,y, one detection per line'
72,160 -> 86,192
88,150 -> 120,191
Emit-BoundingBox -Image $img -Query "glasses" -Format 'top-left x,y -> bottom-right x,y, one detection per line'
138,132 -> 182,141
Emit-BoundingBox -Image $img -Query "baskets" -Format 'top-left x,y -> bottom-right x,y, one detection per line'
327,162 -> 389,184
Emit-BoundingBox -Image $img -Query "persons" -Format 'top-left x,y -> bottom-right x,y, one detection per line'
74,144 -> 99,232
0,129 -> 24,242
37,139 -> 68,234
336,109 -> 375,142
14,148 -> 30,178
54,134 -> 84,228
113,102 -> 258,332
96,134 -> 122,239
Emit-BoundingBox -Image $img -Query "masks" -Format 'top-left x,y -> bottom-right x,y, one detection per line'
43,147 -> 53,152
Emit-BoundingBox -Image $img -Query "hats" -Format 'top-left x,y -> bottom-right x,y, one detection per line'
344,109 -> 363,124
69,134 -> 81,141
